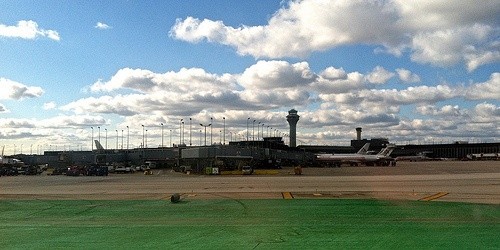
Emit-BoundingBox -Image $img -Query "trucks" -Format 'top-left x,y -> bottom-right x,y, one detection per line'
242,166 -> 253,175
2,158 -> 24,164
0,160 -> 157,176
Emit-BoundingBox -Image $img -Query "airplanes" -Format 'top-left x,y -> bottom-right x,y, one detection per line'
395,151 -> 442,162
312,142 -> 400,168
471,153 -> 500,161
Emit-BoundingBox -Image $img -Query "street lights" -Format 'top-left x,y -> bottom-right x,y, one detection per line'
145,129 -> 147,148
220,131 -> 221,144
91,126 -> 94,157
141,125 -> 145,165
230,117 -> 288,146
160,123 -> 164,159
211,118 -> 213,146
115,126 -> 129,153
105,128 -> 107,153
190,118 -> 192,147
223,117 -> 225,145
98,126 -> 100,155
179,119 -> 185,147
170,130 -> 171,148
200,124 -> 212,147
